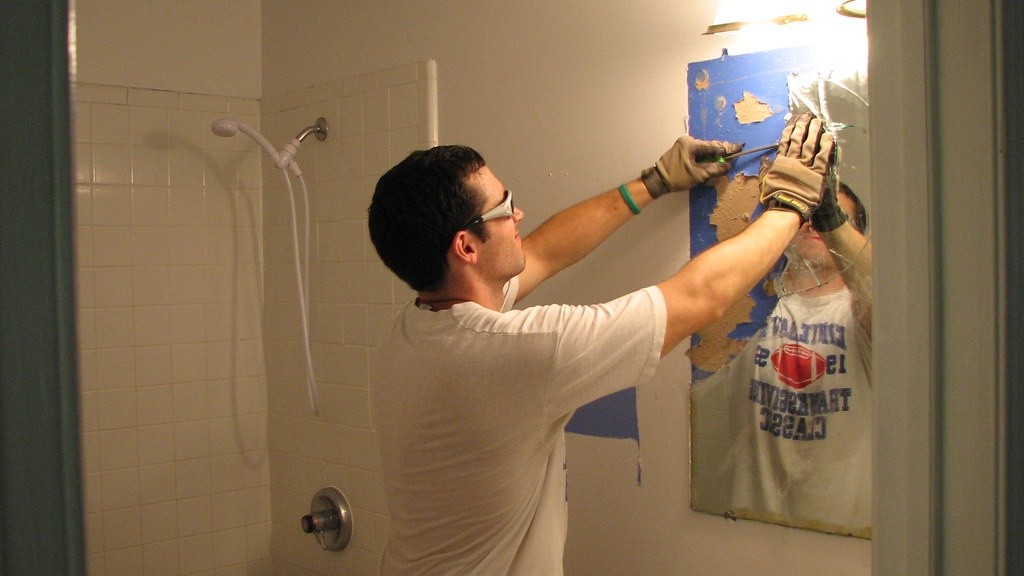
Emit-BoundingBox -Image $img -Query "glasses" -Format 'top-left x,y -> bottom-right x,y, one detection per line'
468,189 -> 514,225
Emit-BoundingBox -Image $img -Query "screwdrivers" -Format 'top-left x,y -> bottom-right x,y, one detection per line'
698,142 -> 780,162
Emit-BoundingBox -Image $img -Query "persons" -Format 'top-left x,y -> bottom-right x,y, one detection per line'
725,142 -> 871,527
370,112 -> 835,576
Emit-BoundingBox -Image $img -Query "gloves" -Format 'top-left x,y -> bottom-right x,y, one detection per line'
760,111 -> 833,225
641,135 -> 744,200
811,143 -> 850,230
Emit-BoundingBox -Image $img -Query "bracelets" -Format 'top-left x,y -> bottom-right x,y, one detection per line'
618,183 -> 641,214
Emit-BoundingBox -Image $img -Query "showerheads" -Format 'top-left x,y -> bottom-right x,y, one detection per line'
211,118 -> 279,162
